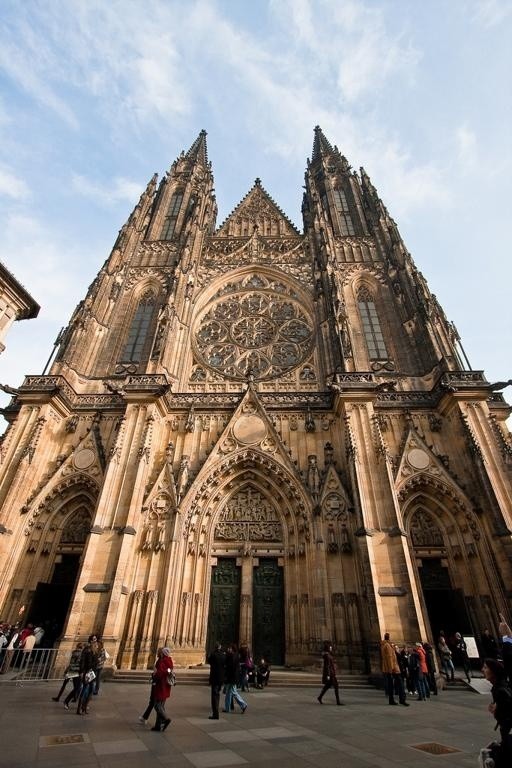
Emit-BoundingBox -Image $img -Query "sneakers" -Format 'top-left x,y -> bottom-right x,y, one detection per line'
317,696 -> 346,706
445,676 -> 471,683
389,698 -> 398,706
208,703 -> 249,721
137,716 -> 172,733
409,688 -> 438,702
399,698 -> 410,707
50,691 -> 99,711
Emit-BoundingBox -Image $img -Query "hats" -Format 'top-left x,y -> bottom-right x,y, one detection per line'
161,647 -> 171,656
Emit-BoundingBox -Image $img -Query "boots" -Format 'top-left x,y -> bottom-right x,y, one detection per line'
76,696 -> 89,716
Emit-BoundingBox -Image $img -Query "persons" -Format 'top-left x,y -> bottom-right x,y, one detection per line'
317,640 -> 345,705
208,640 -> 270,720
0,620 -> 44,670
380,621 -> 512,767
52,635 -> 105,715
139,648 -> 173,732
